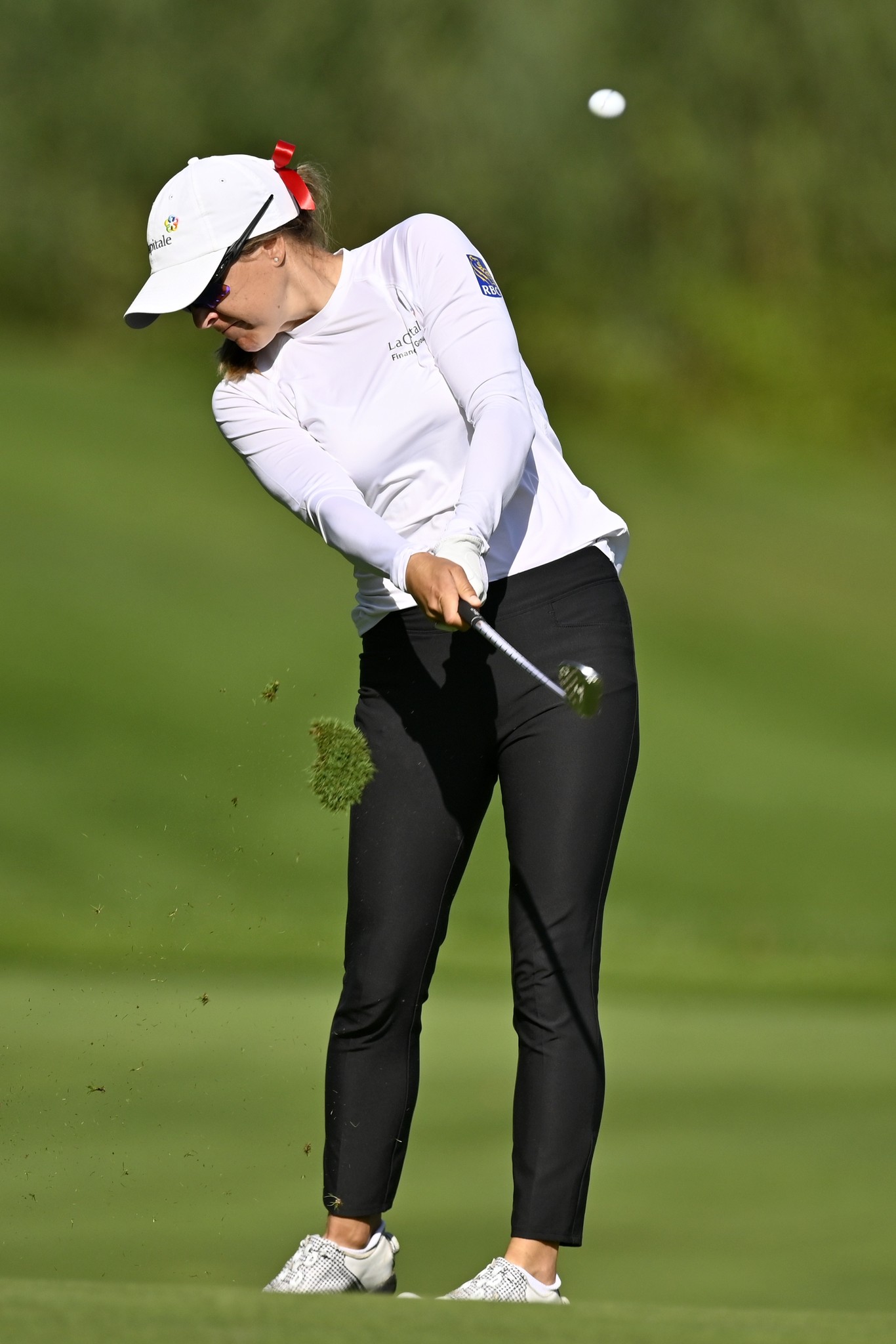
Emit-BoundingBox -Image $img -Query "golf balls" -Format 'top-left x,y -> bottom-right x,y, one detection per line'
587,87 -> 626,119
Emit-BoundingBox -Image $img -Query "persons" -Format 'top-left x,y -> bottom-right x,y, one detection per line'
119,135 -> 644,1306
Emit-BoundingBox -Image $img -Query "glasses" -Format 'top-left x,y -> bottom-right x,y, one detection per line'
182,194 -> 273,314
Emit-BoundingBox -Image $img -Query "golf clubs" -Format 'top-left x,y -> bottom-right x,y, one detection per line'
458,595 -> 602,720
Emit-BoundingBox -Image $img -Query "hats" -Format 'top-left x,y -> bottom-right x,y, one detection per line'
122,154 -> 300,329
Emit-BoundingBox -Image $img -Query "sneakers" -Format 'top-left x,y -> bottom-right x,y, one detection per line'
397,1257 -> 570,1305
263,1219 -> 400,1294
